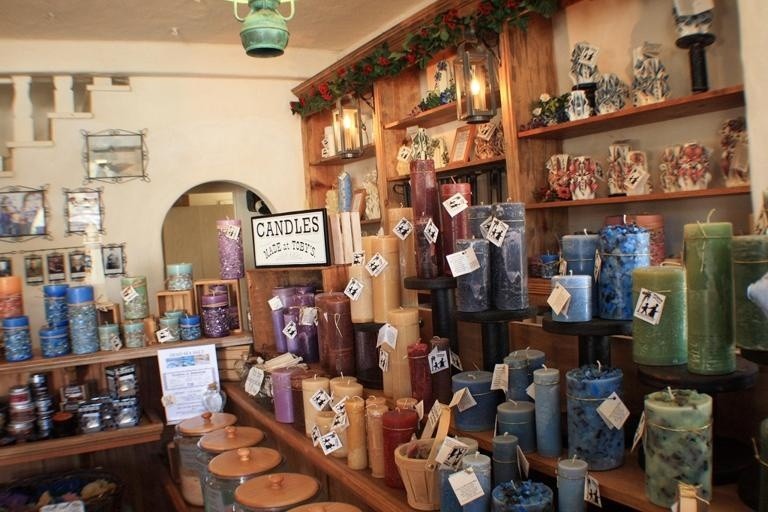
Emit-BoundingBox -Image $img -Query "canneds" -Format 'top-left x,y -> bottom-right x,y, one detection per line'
6,371 -> 56,440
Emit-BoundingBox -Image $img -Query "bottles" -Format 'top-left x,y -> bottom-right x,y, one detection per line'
174,412 -> 361,512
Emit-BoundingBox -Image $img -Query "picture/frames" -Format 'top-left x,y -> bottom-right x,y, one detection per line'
0,184 -> 53,243
61,186 -> 106,239
81,127 -> 151,186
46,252 -> 66,282
100,242 -> 126,278
24,254 -> 43,286
69,251 -> 87,279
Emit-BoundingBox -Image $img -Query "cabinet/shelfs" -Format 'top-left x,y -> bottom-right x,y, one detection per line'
162,1 -> 768,510
0,331 -> 254,468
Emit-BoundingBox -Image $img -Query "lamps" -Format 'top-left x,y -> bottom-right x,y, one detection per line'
452,15 -> 497,123
